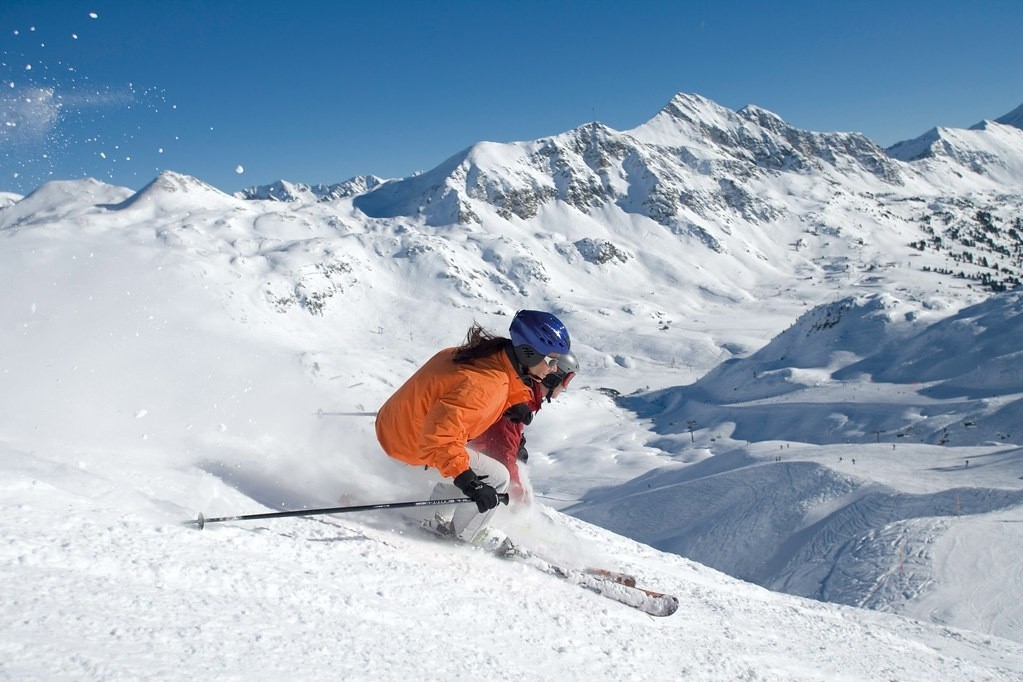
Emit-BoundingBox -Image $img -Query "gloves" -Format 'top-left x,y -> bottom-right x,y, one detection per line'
453,467 -> 499,513
517,438 -> 528,463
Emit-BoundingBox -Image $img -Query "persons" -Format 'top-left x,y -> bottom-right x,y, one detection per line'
466,350 -> 580,514
375,310 -> 570,558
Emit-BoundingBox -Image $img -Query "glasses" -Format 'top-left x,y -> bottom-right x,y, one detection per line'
561,372 -> 576,386
545,356 -> 559,368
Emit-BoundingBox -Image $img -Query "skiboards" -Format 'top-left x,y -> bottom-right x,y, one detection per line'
401,508 -> 681,618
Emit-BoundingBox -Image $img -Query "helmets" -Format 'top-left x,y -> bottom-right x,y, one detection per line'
509,310 -> 570,367
557,350 -> 580,373
